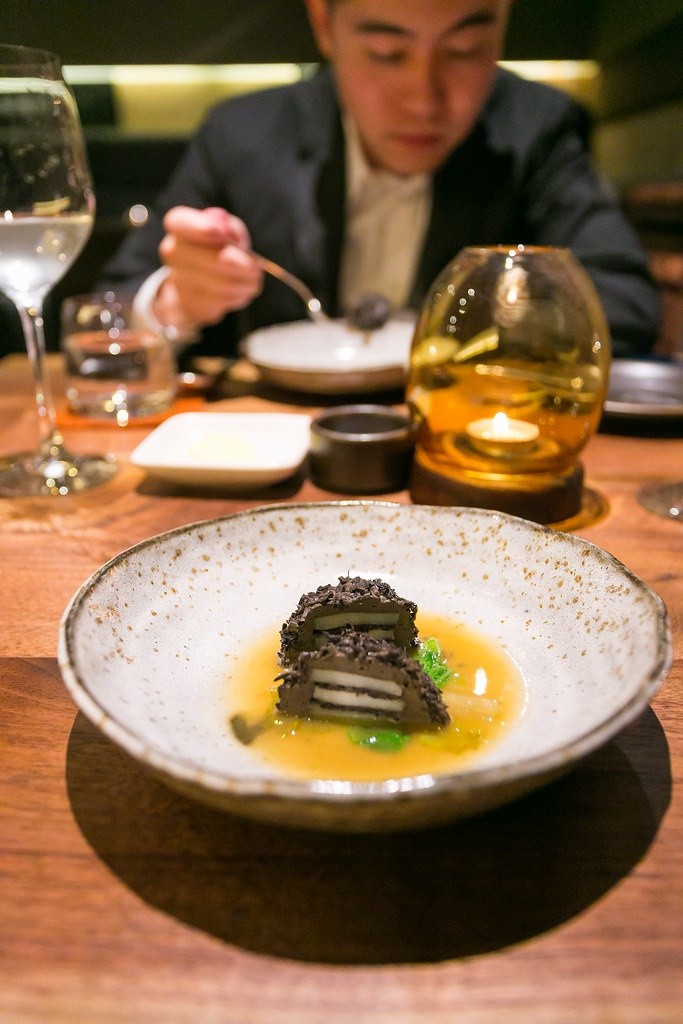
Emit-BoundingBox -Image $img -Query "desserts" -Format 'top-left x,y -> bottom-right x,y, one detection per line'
234,571 -> 527,780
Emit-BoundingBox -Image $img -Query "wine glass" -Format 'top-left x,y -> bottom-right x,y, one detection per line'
0,43 -> 116,499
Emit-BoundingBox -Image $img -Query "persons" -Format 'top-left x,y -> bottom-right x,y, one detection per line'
82,0 -> 659,359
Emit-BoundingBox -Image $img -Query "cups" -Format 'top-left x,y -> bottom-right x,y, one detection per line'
310,406 -> 408,495
64,296 -> 171,417
408,248 -> 610,526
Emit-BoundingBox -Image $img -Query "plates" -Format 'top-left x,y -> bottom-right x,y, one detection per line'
59,500 -> 673,827
603,361 -> 683,414
130,413 -> 310,488
242,319 -> 406,395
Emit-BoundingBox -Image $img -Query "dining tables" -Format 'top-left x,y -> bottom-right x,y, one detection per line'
0,352 -> 683,1024
